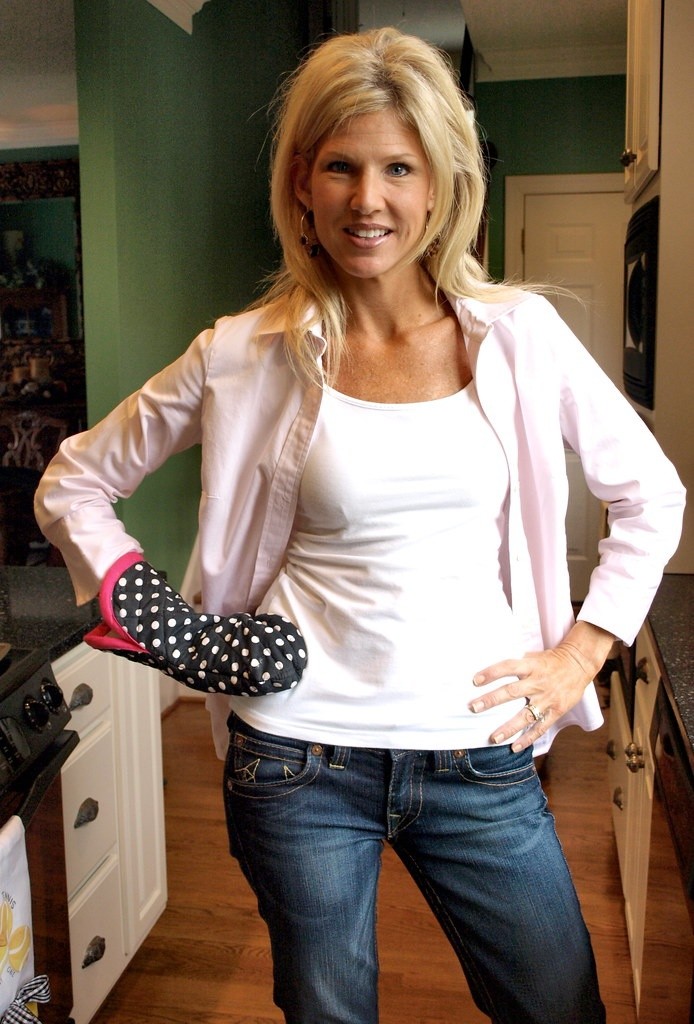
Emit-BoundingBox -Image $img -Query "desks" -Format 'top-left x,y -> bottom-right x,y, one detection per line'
0,465 -> 46,568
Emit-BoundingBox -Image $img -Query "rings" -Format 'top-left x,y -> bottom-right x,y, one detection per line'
525,704 -> 541,721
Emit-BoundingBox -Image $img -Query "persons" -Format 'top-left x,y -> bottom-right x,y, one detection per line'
33,26 -> 687,1024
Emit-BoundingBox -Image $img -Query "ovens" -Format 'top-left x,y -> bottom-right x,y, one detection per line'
0,662 -> 80,1024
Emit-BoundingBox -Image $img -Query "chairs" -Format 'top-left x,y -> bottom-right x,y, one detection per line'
0,409 -> 67,567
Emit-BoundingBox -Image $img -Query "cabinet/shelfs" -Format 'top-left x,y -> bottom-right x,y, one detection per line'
620,0 -> 664,204
607,671 -> 634,890
51,644 -> 170,1024
622,624 -> 663,1015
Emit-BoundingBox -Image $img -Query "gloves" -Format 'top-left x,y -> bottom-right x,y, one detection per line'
83,551 -> 309,698
